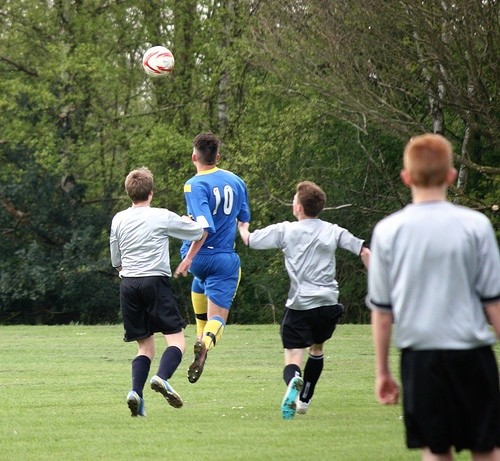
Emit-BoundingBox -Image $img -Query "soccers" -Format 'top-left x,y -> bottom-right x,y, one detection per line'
142,45 -> 175,78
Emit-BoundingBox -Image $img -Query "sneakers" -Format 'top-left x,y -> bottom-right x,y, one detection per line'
187,340 -> 207,383
297,394 -> 313,414
281,376 -> 304,420
126,390 -> 147,417
150,375 -> 183,409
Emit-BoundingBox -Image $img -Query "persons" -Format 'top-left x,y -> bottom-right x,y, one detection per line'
174,131 -> 251,384
239,181 -> 370,419
364,131 -> 500,461
110,165 -> 205,416
